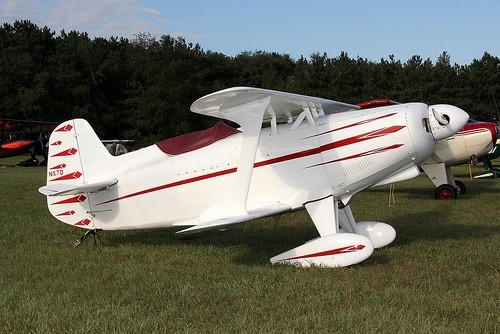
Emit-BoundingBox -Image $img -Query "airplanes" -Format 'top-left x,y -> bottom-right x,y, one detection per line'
416,119 -> 500,200
38,87 -> 469,269
0,118 -> 134,167
